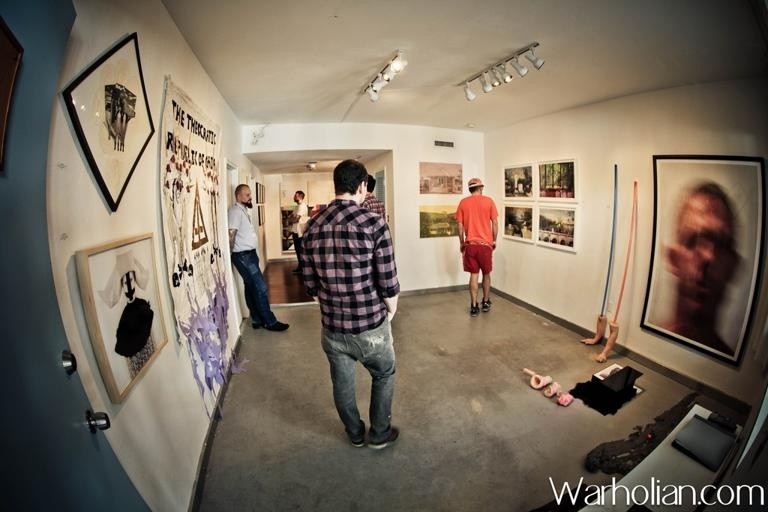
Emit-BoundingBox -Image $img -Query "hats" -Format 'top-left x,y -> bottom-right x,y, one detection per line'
467,177 -> 485,189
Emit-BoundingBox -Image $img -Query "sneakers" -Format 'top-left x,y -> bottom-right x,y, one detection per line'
366,424 -> 402,450
470,302 -> 480,317
261,320 -> 290,331
292,266 -> 303,273
480,295 -> 493,312
350,418 -> 367,448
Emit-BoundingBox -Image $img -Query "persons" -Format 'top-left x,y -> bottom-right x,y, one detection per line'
298,159 -> 401,451
97,250 -> 158,384
648,178 -> 746,356
361,174 -> 386,223
287,191 -> 309,275
582,315 -> 608,347
228,184 -> 290,331
454,177 -> 499,318
597,322 -> 618,363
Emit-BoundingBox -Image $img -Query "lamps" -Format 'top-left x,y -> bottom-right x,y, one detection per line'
359,46 -> 405,106
452,35 -> 547,106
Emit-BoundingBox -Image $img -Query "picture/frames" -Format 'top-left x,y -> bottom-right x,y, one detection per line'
636,151 -> 768,371
499,156 -> 580,255
53,28 -> 161,216
0,15 -> 24,174
69,226 -> 173,408
255,179 -> 267,228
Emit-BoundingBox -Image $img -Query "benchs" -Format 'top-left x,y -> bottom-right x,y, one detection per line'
570,398 -> 738,511
